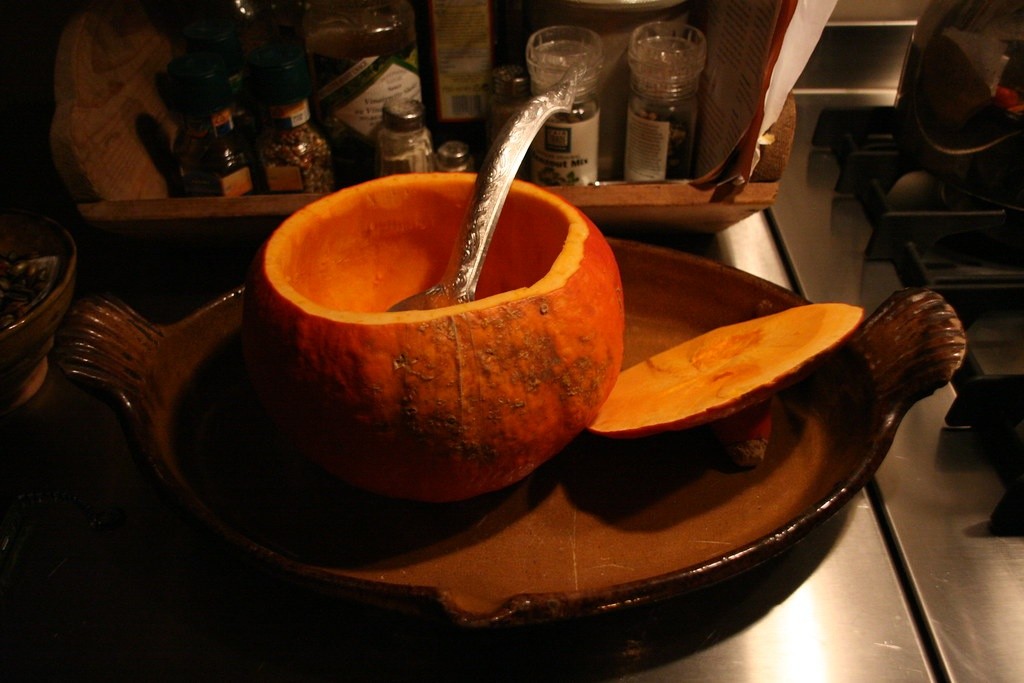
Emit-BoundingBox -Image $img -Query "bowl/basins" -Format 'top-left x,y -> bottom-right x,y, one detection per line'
2,205 -> 76,409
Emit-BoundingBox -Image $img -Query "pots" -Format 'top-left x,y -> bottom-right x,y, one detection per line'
47,235 -> 970,671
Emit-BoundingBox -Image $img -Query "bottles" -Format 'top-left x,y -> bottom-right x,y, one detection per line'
248,42 -> 334,194
524,25 -> 605,185
303,4 -> 425,157
433,139 -> 481,175
181,19 -> 261,145
166,51 -> 262,198
378,100 -> 433,177
488,65 -> 533,184
624,19 -> 708,181
